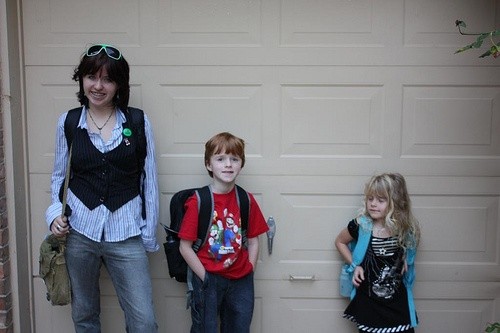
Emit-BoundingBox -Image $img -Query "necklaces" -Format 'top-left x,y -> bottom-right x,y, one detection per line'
88,108 -> 114,130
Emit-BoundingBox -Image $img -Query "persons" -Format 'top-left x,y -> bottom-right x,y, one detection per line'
179,131 -> 270,333
46,45 -> 160,333
335,172 -> 419,333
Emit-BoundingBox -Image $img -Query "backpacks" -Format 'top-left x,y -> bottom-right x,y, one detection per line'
160,183 -> 251,282
339,213 -> 417,297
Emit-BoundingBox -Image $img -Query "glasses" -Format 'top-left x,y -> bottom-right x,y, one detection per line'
81,44 -> 122,60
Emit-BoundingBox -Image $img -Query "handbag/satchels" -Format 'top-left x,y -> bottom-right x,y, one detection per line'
39,233 -> 72,306
